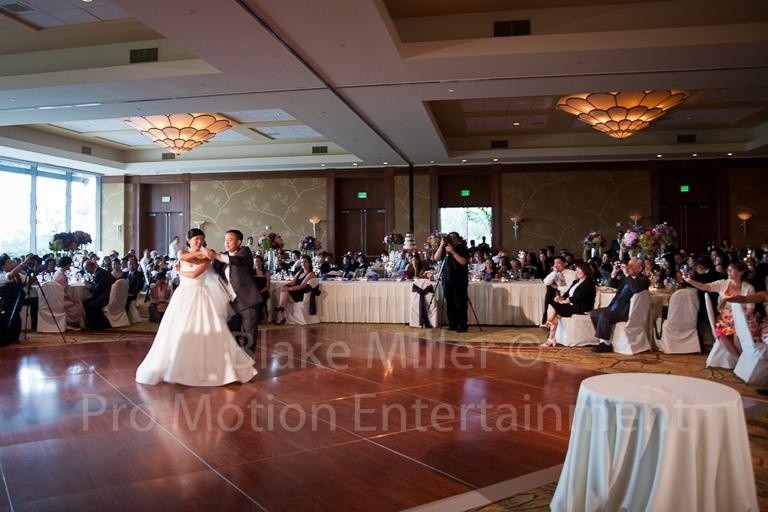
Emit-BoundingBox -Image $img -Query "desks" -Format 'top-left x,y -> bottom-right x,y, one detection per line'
270,279 -> 615,327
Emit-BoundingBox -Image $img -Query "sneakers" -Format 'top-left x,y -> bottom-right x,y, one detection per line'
756,388 -> 768,395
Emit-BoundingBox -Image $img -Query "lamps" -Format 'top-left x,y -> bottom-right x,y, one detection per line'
122,113 -> 239,155
553,89 -> 693,140
739,215 -> 752,238
511,217 -> 522,239
630,216 -> 642,225
309,219 -> 321,236
114,222 -> 124,239
195,221 -> 205,229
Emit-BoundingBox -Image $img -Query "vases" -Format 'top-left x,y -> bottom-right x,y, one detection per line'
590,248 -> 603,259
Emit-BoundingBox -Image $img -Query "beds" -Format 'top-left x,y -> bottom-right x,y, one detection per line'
549,372 -> 761,511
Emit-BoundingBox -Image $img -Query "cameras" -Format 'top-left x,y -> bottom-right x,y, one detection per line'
680,264 -> 693,275
443,234 -> 453,247
25,253 -> 42,268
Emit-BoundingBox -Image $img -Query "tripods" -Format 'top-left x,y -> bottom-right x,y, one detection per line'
421,254 -> 483,336
3,270 -> 67,343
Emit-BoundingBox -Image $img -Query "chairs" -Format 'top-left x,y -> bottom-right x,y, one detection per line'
553,285 -> 619,347
610,290 -> 652,355
730,302 -> 767,386
409,279 -> 438,328
652,289 -> 702,356
705,293 -> 738,368
13,279 -> 142,333
284,278 -> 320,325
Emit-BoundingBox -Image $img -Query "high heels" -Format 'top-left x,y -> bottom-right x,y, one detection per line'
275,317 -> 286,325
273,305 -> 284,314
540,321 -> 555,330
540,337 -> 556,348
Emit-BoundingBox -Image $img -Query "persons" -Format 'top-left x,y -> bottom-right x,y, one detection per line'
202,229 -> 263,358
135,228 -> 258,387
246,231 -> 768,354
0,236 -> 223,348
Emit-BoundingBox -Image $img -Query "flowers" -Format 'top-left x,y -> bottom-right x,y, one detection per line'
383,233 -> 404,245
622,223 -> 677,261
583,231 -> 606,248
299,235 -> 322,251
258,232 -> 283,251
48,230 -> 91,250
425,230 -> 448,251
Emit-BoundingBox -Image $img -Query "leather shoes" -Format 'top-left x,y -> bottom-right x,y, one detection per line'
67,325 -> 81,331
591,342 -> 613,352
